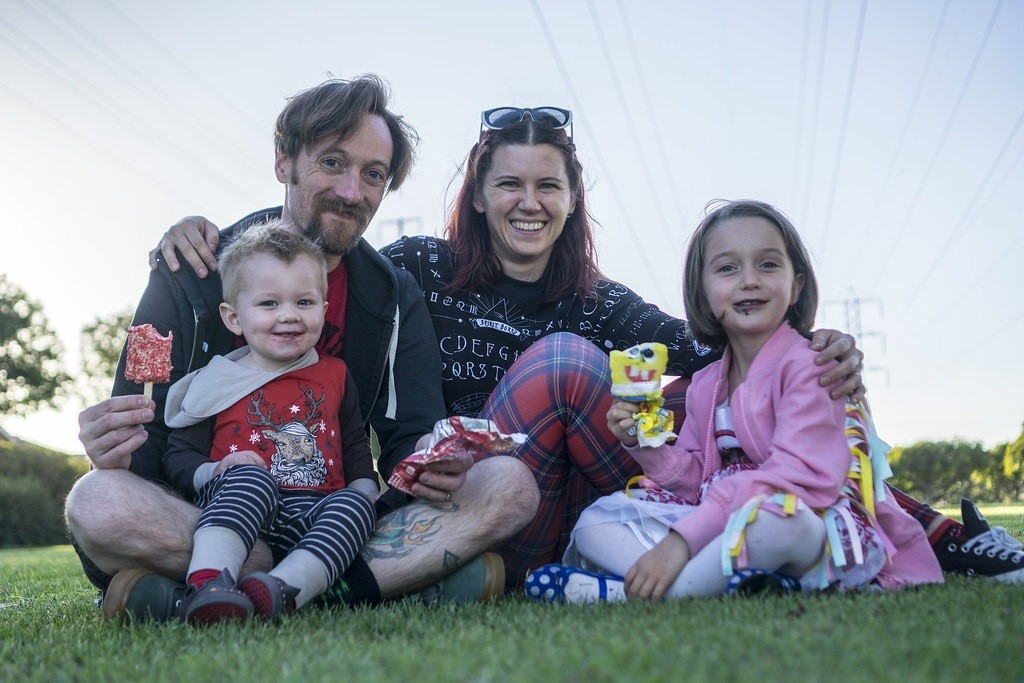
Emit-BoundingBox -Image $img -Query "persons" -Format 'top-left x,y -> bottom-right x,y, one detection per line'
64,68 -> 542,625
163,212 -> 381,630
147,107 -> 1024,594
523,196 -> 946,608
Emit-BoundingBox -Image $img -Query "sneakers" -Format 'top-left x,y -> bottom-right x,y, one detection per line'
101,565 -> 188,626
405,551 -> 506,604
176,571 -> 299,626
933,498 -> 1024,581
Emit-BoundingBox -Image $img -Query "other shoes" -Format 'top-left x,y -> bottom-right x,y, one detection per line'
524,560 -> 626,607
726,568 -> 802,600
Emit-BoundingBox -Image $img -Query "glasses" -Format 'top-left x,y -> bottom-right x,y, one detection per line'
479,106 -> 575,146
